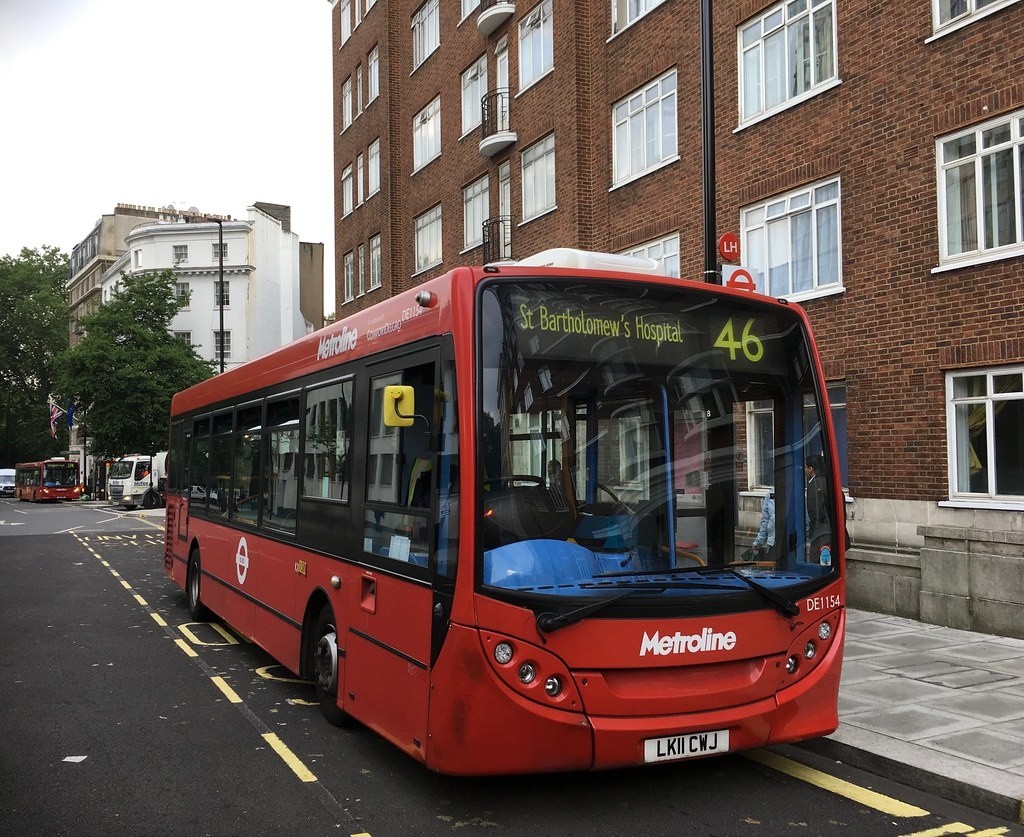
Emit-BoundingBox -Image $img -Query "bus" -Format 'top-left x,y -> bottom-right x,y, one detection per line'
163,247 -> 847,779
15,457 -> 83,503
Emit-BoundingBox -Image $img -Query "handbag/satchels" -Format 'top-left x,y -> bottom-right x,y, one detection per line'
741,547 -> 767,561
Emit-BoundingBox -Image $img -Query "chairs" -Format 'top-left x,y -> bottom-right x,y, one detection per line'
404,445 -> 492,535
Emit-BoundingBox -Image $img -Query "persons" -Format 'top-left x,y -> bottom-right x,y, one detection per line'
547,460 -> 563,488
751,454 -> 851,574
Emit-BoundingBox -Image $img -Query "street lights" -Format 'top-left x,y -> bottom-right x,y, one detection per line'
206,218 -> 226,373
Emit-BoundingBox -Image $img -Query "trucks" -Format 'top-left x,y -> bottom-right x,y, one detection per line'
108,452 -> 170,511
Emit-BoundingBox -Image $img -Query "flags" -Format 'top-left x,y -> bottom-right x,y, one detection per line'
66,399 -> 79,430
48,402 -> 64,438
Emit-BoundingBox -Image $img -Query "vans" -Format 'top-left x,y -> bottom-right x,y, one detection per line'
0,469 -> 17,497
